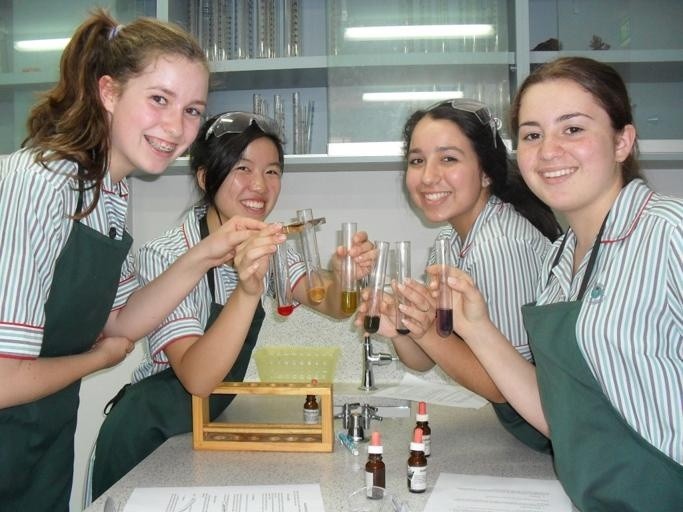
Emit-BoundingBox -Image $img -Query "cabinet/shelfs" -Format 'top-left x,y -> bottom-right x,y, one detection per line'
0,0 -> 683,161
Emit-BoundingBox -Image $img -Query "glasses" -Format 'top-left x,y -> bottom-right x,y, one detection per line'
421,98 -> 495,133
206,112 -> 278,138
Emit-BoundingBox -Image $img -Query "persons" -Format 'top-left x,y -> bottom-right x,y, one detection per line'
91,111 -> 381,498
426,56 -> 682,511
356,98 -> 557,452
1,9 -> 208,509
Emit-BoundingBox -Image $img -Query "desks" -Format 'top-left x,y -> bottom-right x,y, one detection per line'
82,382 -> 579,512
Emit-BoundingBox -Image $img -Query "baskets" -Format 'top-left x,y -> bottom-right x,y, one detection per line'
253,346 -> 338,382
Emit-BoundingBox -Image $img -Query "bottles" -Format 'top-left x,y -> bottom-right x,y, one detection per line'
296,209 -> 326,303
406,427 -> 429,494
348,413 -> 364,442
362,240 -> 390,334
268,221 -> 293,317
364,431 -> 386,500
293,91 -> 315,154
338,221 -> 359,318
187,0 -> 303,62
412,401 -> 432,458
392,241 -> 412,336
434,238 -> 454,339
252,93 -> 269,116
302,379 -> 320,425
273,95 -> 287,155
